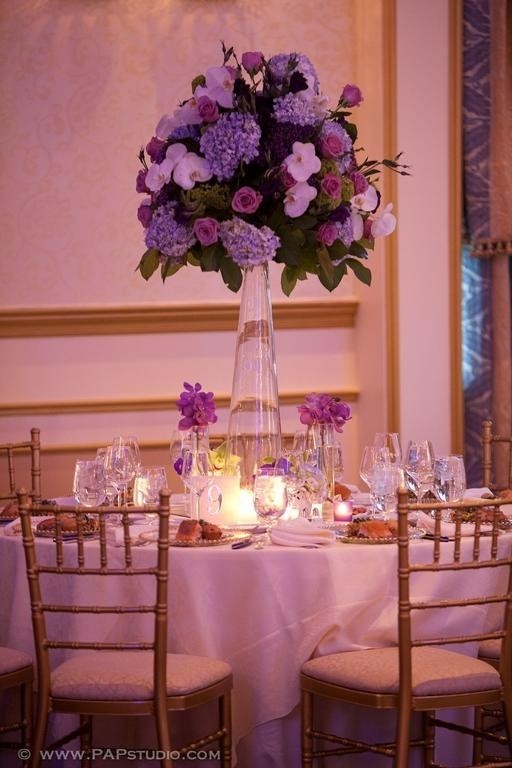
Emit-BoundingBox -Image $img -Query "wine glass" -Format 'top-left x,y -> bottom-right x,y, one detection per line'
71,428 -> 469,547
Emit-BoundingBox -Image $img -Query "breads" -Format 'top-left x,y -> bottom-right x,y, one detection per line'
347,518 -> 398,538
176,519 -> 222,541
37,517 -> 100,530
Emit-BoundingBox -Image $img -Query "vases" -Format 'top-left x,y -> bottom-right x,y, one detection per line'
224,259 -> 283,492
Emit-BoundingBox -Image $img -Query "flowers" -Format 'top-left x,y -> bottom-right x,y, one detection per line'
134,41 -> 413,298
296,391 -> 352,434
175,381 -> 218,431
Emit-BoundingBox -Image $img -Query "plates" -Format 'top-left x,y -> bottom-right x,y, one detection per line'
0,505 -> 48,522
31,522 -> 100,537
448,515 -> 512,532
